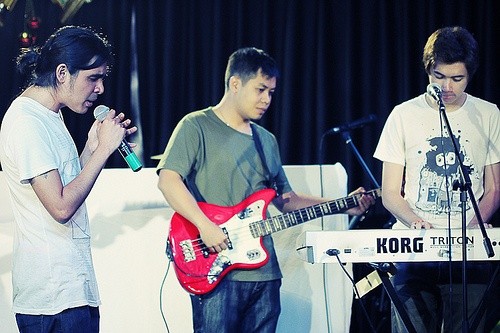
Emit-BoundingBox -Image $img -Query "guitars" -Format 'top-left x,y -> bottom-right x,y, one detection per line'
167,187 -> 382,295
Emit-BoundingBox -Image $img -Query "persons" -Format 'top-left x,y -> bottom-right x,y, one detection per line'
157,48 -> 375,333
0,26 -> 137,333
373,26 -> 500,333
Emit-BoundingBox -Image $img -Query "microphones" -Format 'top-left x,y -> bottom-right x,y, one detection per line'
93,105 -> 143,172
427,83 -> 446,110
330,115 -> 377,134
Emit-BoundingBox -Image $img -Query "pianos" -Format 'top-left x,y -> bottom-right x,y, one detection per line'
295,228 -> 500,264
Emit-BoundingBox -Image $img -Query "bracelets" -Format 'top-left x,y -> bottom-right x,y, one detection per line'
412,220 -> 425,225
